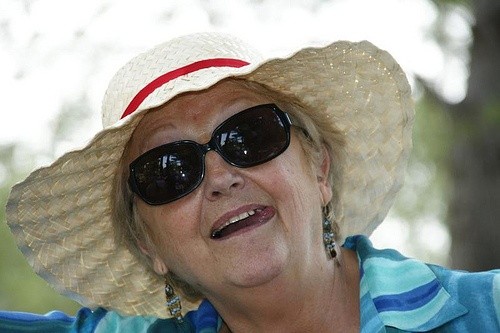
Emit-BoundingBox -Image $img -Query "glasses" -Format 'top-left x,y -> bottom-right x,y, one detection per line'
127,101 -> 313,207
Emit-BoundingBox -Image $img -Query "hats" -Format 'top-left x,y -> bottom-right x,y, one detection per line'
5,29 -> 416,320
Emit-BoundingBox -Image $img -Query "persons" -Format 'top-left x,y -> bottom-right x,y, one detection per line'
0,34 -> 500,333
